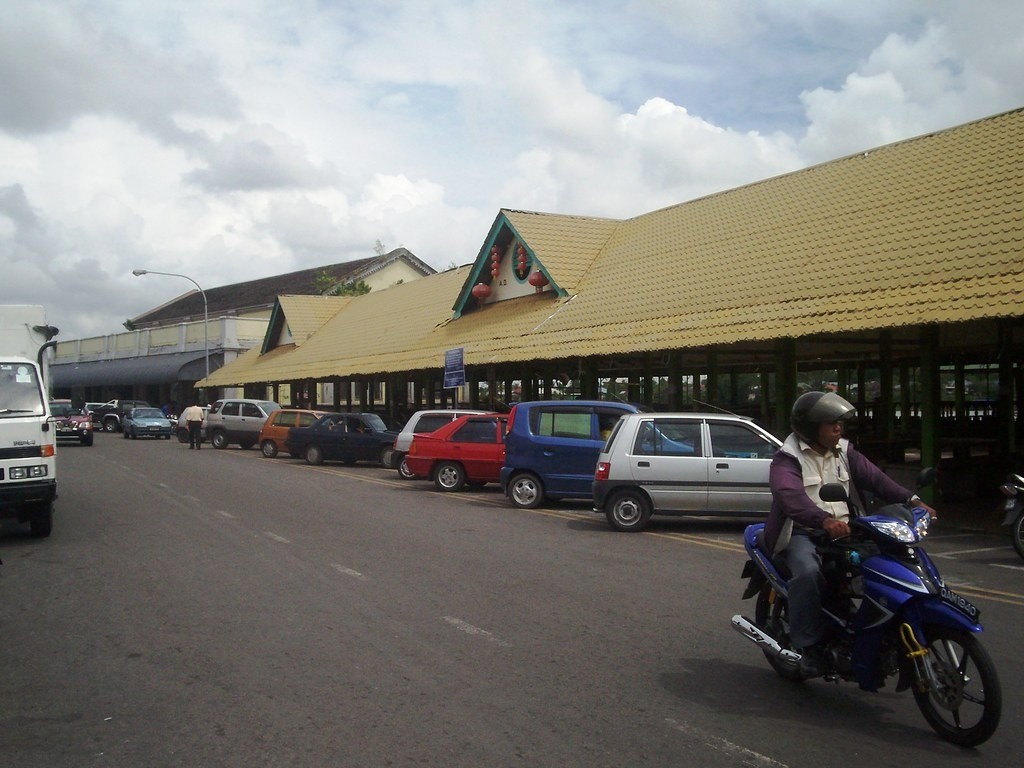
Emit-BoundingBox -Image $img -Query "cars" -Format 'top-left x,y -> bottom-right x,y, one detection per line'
499,401 -> 727,509
175,406 -> 218,443
80,402 -> 108,415
94,399 -> 152,432
284,413 -> 399,469
259,409 -> 330,457
124,408 -> 172,439
206,399 -> 282,449
592,412 -> 784,532
391,409 -> 498,479
49,399 -> 94,446
405,414 -> 509,492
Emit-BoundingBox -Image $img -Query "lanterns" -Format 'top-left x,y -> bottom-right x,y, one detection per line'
491,244 -> 500,280
472,283 -> 491,305
528,272 -> 550,295
517,241 -> 527,277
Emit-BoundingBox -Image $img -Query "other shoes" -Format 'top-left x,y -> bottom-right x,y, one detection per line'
800,643 -> 823,678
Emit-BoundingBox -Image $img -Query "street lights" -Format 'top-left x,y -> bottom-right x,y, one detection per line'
133,270 -> 209,404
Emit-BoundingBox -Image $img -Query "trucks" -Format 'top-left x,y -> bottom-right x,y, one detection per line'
0,359 -> 56,537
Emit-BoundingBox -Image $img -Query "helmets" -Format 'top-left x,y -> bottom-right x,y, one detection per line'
790,391 -> 842,444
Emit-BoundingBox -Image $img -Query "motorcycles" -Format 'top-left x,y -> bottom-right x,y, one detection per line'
1001,473 -> 1024,559
731,507 -> 1003,747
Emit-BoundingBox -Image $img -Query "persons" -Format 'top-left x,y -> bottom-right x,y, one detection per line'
185,398 -> 204,450
764,392 -> 937,678
